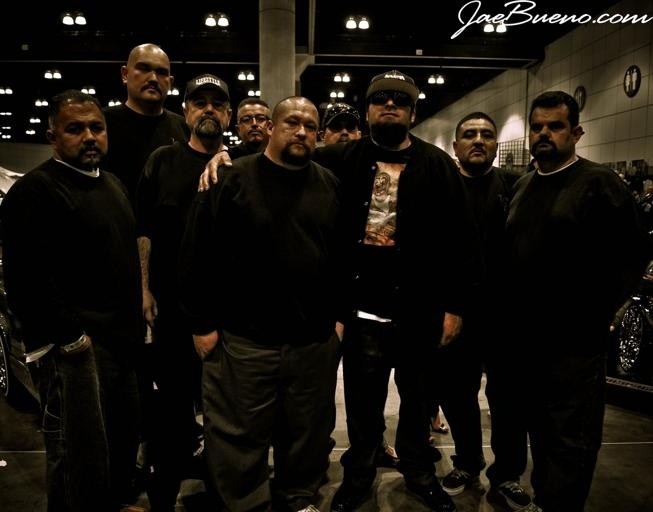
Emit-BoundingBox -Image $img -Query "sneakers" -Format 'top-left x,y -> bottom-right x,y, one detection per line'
298,417 -> 530,512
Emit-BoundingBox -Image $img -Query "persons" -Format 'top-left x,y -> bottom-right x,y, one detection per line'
0,42 -> 651,511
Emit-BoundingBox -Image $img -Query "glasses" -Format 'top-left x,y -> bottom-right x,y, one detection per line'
370,91 -> 413,108
328,120 -> 355,132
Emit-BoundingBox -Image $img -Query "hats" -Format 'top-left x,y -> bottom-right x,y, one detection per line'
322,103 -> 360,130
367,70 -> 419,106
184,74 -> 229,102
